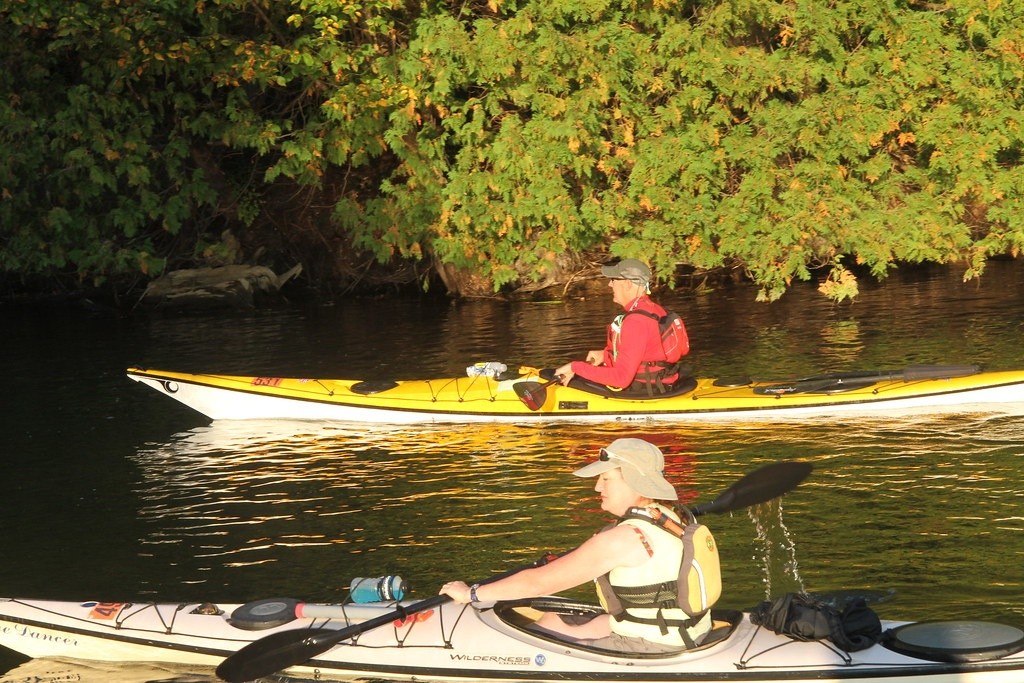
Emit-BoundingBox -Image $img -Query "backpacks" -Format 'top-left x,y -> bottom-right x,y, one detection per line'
749,592 -> 883,652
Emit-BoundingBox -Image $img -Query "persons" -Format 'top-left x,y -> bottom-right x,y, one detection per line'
438,437 -> 713,654
553,258 -> 680,397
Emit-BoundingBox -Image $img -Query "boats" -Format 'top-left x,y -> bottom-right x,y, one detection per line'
126,365 -> 1024,422
1,587 -> 1022,674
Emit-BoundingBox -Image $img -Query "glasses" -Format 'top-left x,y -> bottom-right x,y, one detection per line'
599,448 -> 646,476
611,277 -> 640,282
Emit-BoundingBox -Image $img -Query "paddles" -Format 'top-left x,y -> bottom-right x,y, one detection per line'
512,356 -> 597,412
214,460 -> 815,683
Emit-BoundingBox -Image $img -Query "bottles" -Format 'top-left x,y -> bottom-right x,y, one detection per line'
350,575 -> 410,604
466,362 -> 507,377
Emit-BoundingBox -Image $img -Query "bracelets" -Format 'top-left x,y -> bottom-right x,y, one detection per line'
470,583 -> 482,603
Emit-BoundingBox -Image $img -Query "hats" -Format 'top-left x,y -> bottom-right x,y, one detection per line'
572,437 -> 678,501
601,258 -> 652,286
